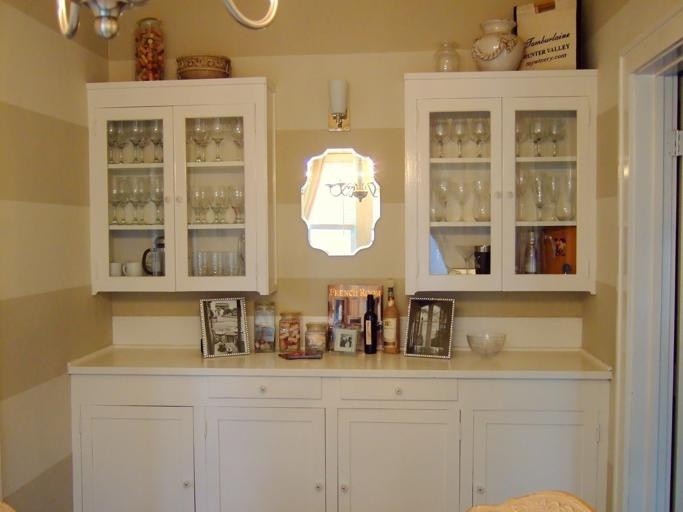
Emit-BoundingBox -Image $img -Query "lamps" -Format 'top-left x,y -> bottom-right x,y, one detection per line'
328,78 -> 351,131
324,159 -> 378,202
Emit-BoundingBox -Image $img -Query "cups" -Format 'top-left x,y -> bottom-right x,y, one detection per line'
109,263 -> 121,276
473,246 -> 490,275
192,250 -> 242,274
122,260 -> 141,276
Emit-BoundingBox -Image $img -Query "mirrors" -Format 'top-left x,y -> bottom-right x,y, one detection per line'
301,147 -> 381,257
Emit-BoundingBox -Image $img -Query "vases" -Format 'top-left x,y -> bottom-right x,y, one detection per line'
471,19 -> 525,71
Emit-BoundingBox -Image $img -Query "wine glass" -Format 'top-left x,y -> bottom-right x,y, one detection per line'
429,112 -> 489,222
185,118 -> 245,224
105,120 -> 164,226
516,115 -> 575,221
454,243 -> 475,276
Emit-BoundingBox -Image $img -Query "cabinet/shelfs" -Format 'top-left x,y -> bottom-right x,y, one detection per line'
403,69 -> 596,296
86,76 -> 277,295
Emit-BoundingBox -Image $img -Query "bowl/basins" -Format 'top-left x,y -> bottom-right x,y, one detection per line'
466,334 -> 506,357
173,54 -> 230,78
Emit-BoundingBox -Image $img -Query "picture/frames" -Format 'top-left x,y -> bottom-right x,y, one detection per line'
199,296 -> 250,358
403,297 -> 456,359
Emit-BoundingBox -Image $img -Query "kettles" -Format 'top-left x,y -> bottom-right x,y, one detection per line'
141,236 -> 164,276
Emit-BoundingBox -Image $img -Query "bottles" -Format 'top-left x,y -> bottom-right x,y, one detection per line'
303,322 -> 327,354
277,311 -> 302,352
131,20 -> 164,80
252,299 -> 275,353
382,285 -> 399,355
523,227 -> 538,275
431,40 -> 459,73
363,293 -> 377,356
